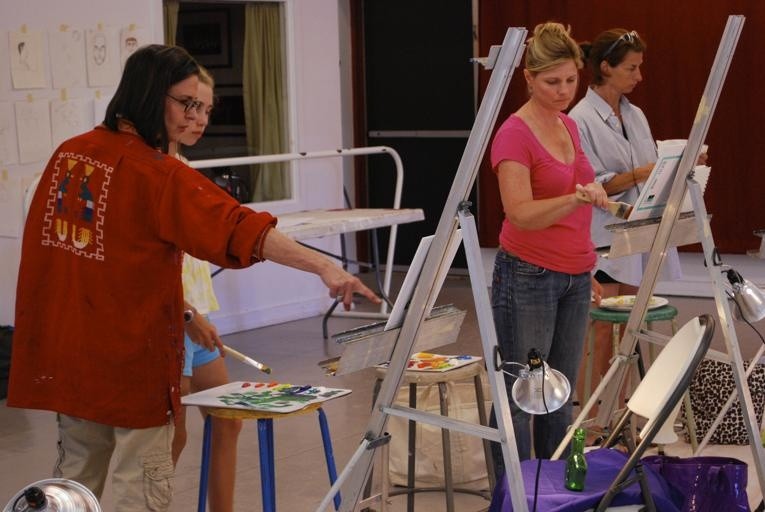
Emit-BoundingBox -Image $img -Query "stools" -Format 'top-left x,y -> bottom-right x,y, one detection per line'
583,307 -> 697,445
199,403 -> 341,511
362,363 -> 497,512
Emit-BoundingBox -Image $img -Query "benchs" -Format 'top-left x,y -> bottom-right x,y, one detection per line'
190,145 -> 425,339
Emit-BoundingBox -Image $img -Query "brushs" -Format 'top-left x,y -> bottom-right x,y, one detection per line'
575,191 -> 634,220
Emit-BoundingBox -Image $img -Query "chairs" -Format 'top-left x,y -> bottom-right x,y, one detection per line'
488,314 -> 715,512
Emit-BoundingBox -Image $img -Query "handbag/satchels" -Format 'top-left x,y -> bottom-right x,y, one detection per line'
674,360 -> 765,446
643,452 -> 751,512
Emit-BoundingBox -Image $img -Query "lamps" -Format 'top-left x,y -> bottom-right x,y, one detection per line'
713,246 -> 765,326
492,345 -> 571,415
2,479 -> 101,511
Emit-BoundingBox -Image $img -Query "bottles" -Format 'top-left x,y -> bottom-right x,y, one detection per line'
564,427 -> 588,492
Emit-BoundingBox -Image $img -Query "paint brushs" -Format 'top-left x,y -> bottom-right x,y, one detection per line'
212,341 -> 272,375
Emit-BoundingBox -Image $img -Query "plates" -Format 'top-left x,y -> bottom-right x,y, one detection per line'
599,294 -> 668,311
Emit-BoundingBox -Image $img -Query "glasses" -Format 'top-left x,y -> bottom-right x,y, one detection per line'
165,93 -> 201,113
602,30 -> 641,59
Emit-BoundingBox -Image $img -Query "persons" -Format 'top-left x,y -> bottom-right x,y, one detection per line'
489,23 -> 609,488
565,28 -> 709,454
6,44 -> 381,511
158,66 -> 244,511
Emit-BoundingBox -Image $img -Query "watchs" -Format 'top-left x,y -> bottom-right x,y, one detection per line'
183,307 -> 196,325
